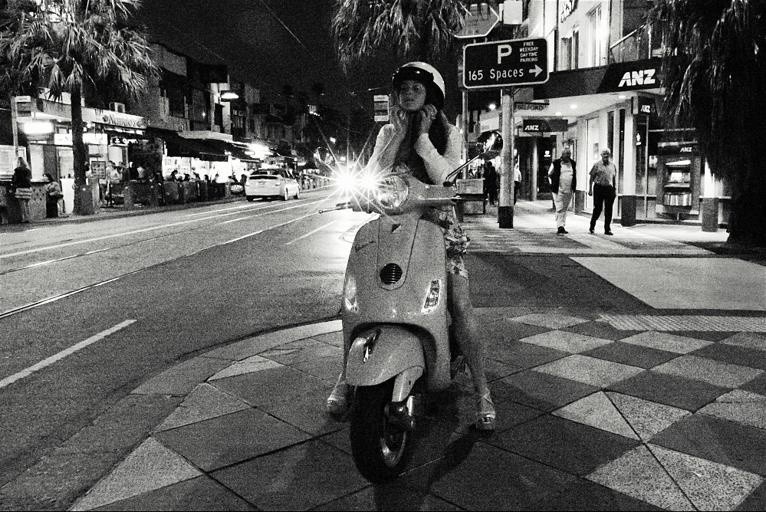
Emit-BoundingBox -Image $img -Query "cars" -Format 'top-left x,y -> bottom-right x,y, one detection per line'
244,166 -> 301,201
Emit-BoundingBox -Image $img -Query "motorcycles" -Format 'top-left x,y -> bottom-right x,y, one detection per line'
314,130 -> 503,487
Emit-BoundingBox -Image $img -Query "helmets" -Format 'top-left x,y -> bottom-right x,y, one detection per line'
393,61 -> 445,106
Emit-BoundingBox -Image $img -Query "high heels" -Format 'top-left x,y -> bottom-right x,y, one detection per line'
476,389 -> 496,429
326,375 -> 350,413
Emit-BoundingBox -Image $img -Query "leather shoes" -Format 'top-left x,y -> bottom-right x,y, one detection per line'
590,227 -> 594,233
604,230 -> 612,235
557,226 -> 568,233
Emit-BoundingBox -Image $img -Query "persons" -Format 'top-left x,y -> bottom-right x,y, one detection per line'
544,147 -> 578,234
484,160 -> 498,207
513,162 -> 520,204
41,172 -> 61,218
588,148 -> 617,235
10,156 -> 33,223
324,60 -> 497,431
